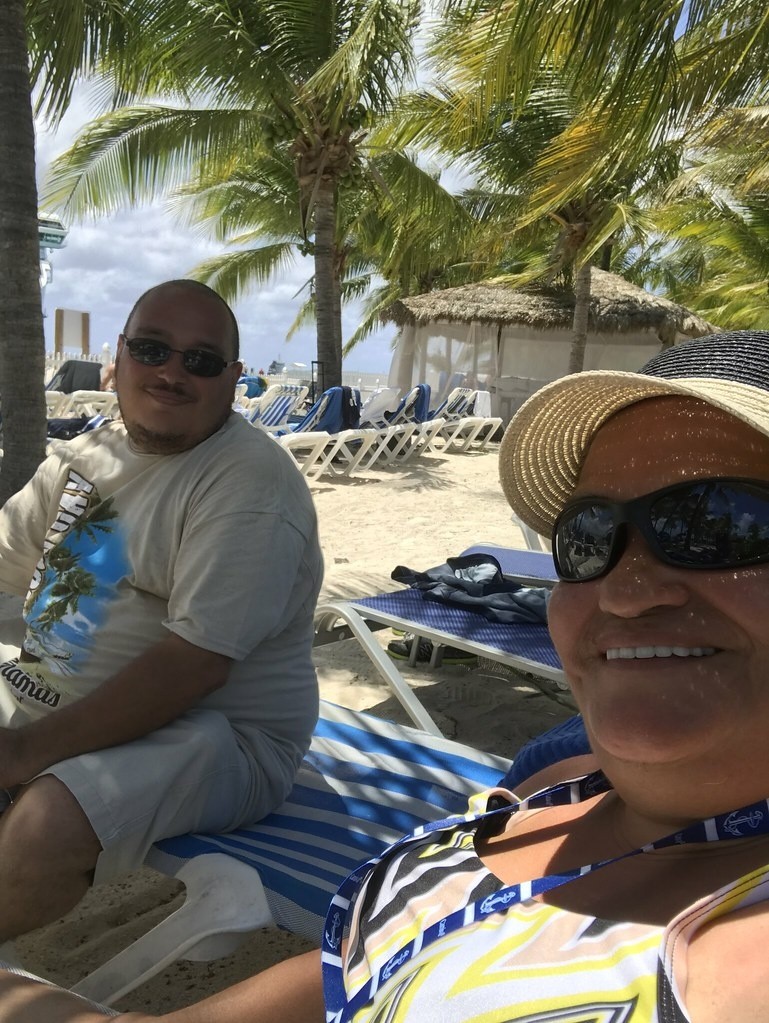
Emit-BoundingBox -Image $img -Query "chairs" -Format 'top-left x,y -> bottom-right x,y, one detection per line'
0,359 -> 574,1014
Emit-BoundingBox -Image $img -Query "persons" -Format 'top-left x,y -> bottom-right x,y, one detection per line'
0,328 -> 769,1022
0,277 -> 324,945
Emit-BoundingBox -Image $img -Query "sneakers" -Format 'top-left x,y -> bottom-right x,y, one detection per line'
387,627 -> 479,665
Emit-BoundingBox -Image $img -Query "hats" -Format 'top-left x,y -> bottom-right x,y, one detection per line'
498,329 -> 769,541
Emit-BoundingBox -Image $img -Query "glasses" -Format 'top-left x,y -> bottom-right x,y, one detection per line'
551,476 -> 769,584
120,334 -> 239,379
